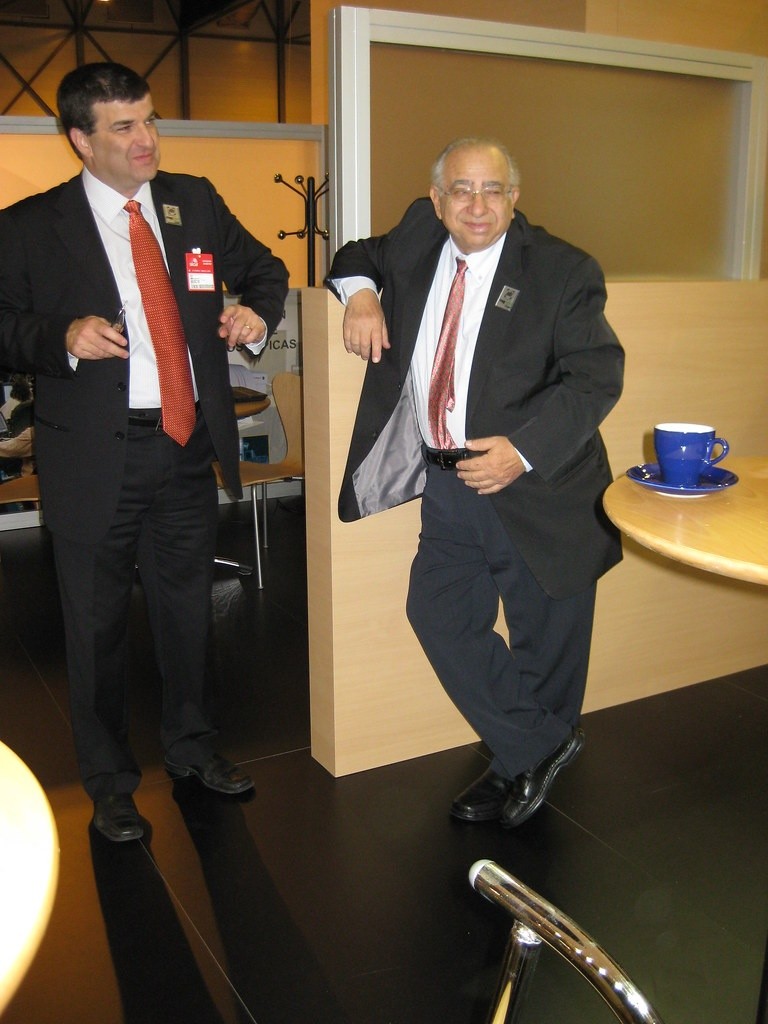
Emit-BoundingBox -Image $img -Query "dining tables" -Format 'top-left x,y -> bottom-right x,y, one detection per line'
601,458 -> 768,592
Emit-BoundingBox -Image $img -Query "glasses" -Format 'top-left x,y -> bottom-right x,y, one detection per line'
109,307 -> 131,338
433,185 -> 521,203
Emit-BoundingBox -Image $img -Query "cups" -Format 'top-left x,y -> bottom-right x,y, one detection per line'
653,422 -> 728,489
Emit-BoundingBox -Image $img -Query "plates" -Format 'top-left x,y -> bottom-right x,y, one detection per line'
625,462 -> 740,499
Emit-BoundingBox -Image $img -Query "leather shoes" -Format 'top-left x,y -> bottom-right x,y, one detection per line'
163,750 -> 256,796
92,793 -> 146,841
451,765 -> 512,823
501,728 -> 587,831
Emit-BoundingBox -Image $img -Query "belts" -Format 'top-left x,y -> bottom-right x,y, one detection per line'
124,413 -> 206,431
418,443 -> 484,471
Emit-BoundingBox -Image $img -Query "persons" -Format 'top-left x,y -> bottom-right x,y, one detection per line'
323,133 -> 630,828
1,60 -> 292,840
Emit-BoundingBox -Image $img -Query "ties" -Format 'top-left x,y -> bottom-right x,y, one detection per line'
427,256 -> 471,448
123,200 -> 197,446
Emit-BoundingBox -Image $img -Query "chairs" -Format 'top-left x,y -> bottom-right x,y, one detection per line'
469,856 -> 663,1023
0,475 -> 41,511
209,372 -> 305,589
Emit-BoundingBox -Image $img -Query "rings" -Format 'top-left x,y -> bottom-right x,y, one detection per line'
244,325 -> 252,331
